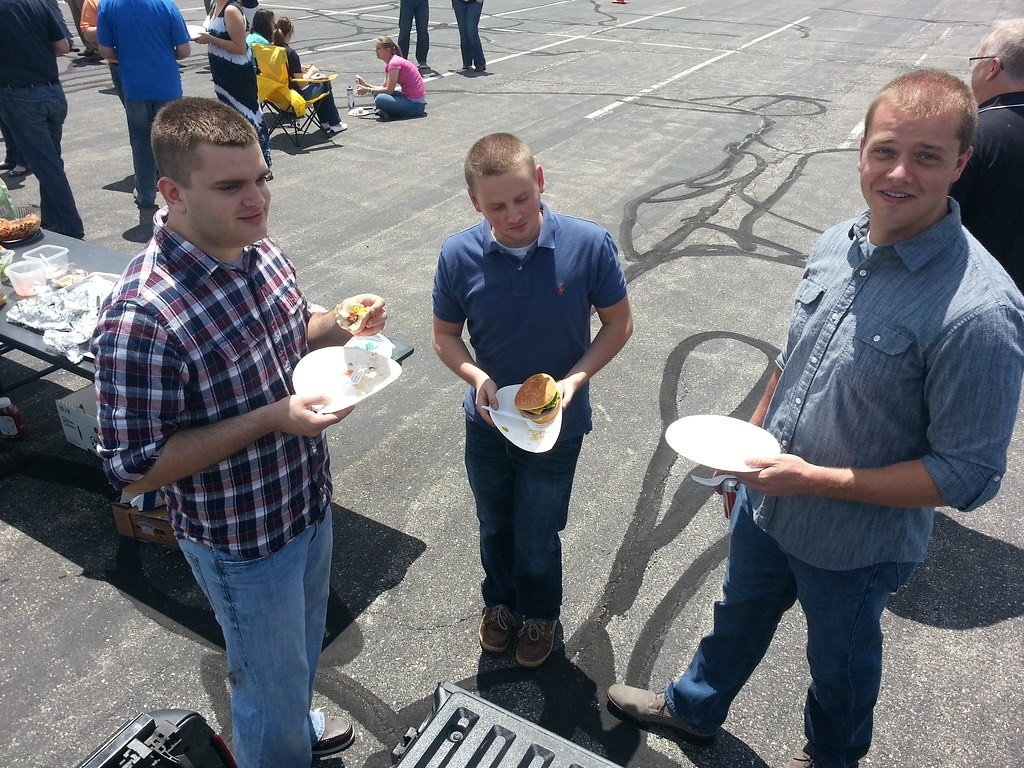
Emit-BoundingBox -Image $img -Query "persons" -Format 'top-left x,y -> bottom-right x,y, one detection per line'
195,0 -> 348,182
397,0 -> 486,72
355,36 -> 427,120
0,0 -> 86,240
65,0 -> 191,236
91,95 -> 386,768
949,17 -> 1024,296
607,65 -> 1024,768
432,133 -> 634,666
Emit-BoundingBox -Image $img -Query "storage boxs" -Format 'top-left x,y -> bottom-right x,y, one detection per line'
57,383 -> 106,460
112,494 -> 184,549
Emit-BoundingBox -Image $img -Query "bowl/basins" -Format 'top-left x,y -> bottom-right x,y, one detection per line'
22,244 -> 69,279
4,259 -> 47,297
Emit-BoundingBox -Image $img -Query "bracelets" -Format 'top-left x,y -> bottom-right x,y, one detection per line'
371,87 -> 372,94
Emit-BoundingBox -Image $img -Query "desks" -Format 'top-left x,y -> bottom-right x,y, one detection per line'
0,214 -> 415,394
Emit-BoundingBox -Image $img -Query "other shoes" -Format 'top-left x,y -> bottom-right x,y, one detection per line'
78,50 -> 92,56
380,112 -> 389,119
330,122 -> 347,132
460,64 -> 471,73
0,161 -> 16,170
7,167 -> 31,178
321,123 -> 330,129
87,54 -> 102,60
475,63 -> 486,72
312,716 -> 355,755
418,62 -> 427,68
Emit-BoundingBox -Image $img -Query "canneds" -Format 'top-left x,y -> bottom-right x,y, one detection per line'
721,478 -> 740,520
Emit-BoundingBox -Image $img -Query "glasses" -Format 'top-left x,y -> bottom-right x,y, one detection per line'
968,56 -> 1004,71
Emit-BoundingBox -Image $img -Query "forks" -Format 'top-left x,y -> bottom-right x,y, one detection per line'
317,363 -> 367,415
481,405 -> 551,431
691,474 -> 738,486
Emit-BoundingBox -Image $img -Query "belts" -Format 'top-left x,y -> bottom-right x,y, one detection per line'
4,80 -> 60,89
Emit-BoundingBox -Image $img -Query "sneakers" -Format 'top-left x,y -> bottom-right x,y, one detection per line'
608,683 -> 708,737
784,748 -> 857,768
479,605 -> 515,652
516,618 -> 557,667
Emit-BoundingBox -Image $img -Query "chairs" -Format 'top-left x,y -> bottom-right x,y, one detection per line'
251,43 -> 332,148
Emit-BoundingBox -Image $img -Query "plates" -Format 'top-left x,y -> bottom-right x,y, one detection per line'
186,25 -> 206,39
0,229 -> 44,248
488,384 -> 562,453
292,346 -> 402,414
665,414 -> 781,472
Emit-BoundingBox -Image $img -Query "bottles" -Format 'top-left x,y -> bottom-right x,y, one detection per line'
347,86 -> 354,108
1,397 -> 25,440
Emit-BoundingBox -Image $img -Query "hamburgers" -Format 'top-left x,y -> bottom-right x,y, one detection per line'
515,373 -> 561,423
334,302 -> 372,334
